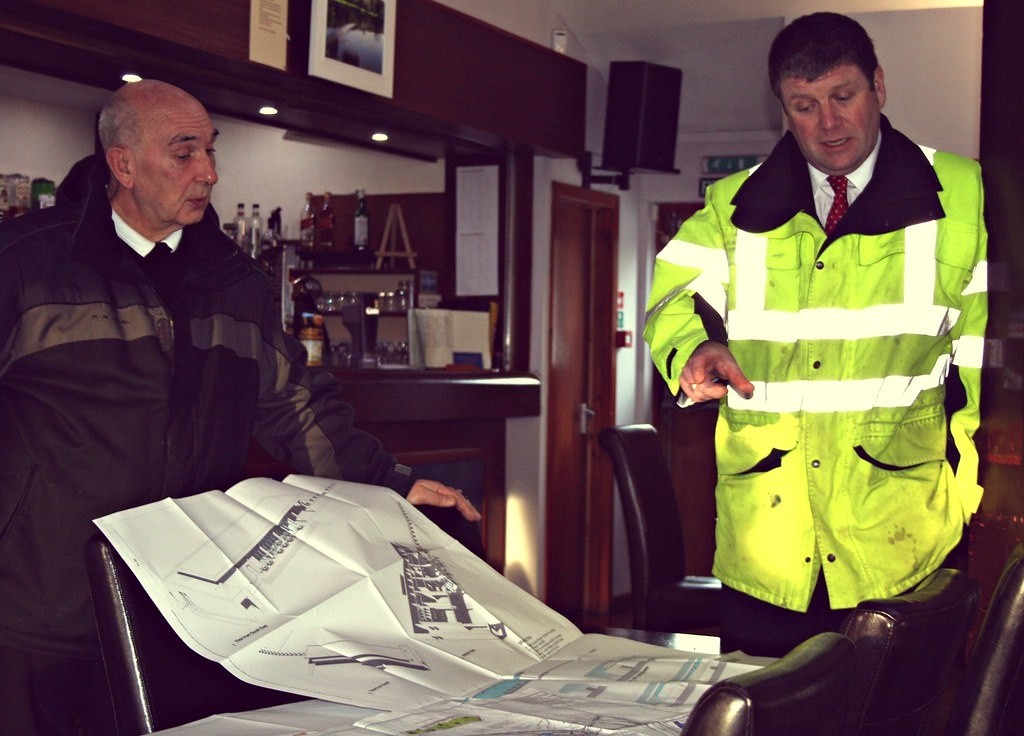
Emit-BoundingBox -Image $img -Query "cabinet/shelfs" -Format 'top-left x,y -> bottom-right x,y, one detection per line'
289,269 -> 420,316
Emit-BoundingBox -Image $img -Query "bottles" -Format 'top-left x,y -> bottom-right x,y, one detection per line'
299,328 -> 323,366
299,192 -> 316,239
224,201 -> 281,267
1,174 -> 56,220
314,280 -> 409,313
316,191 -> 336,247
354,189 -> 370,251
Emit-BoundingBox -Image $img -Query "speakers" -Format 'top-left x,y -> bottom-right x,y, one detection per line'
601,60 -> 682,173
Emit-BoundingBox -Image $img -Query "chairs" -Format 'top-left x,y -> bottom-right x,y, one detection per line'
598,423 -> 722,630
83,505 -> 488,736
681,543 -> 1024,736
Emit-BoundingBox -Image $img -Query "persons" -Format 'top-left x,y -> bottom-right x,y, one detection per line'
0,82 -> 481,736
642,13 -> 990,660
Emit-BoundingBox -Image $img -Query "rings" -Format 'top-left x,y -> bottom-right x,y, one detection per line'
690,383 -> 696,391
